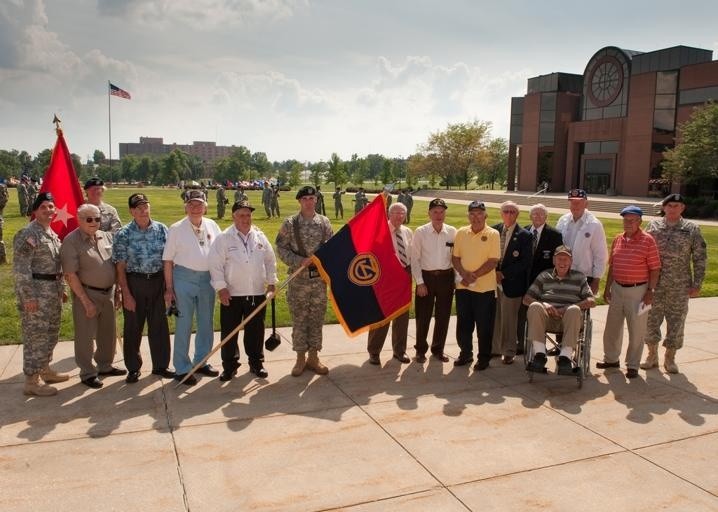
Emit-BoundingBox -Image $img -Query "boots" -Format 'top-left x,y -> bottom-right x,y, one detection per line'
306,349 -> 328,375
41,365 -> 69,383
22,374 -> 57,397
291,351 -> 306,377
640,343 -> 659,369
663,348 -> 679,374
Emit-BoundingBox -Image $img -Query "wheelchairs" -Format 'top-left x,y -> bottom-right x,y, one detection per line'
523,306 -> 593,388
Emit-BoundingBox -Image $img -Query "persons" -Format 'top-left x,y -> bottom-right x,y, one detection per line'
203,186 -> 208,215
488,201 -> 532,364
217,184 -> 228,219
594,206 -> 661,378
262,181 -> 273,219
61,204 -> 127,388
81,177 -> 123,237
240,187 -> 248,202
272,184 -> 281,218
275,186 -> 333,377
17,174 -> 40,217
451,201 -> 500,371
397,189 -> 404,202
315,186 -> 326,216
516,203 -> 562,356
0,183 -> 8,265
162,190 -> 219,386
355,188 -> 368,215
642,194 -> 706,373
522,245 -> 596,375
207,202 -> 279,381
234,187 -> 241,201
113,193 -> 176,383
333,187 -> 345,219
368,202 -> 414,365
411,199 -> 457,363
403,190 -> 413,223
13,192 -> 69,397
545,189 -> 608,362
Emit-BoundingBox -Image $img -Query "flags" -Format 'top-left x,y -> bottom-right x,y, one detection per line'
309,193 -> 413,337
33,128 -> 85,242
108,83 -> 131,100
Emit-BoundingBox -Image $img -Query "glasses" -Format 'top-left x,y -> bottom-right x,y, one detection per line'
86,217 -> 101,224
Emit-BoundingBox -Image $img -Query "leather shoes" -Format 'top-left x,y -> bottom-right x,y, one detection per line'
415,351 -> 426,363
557,356 -> 573,375
432,353 -> 449,362
392,351 -> 410,364
249,366 -> 268,378
126,371 -> 141,383
453,356 -> 474,367
175,373 -> 197,386
532,352 -> 548,370
473,359 -> 489,370
626,368 -> 637,379
151,367 -> 176,379
368,354 -> 380,365
220,370 -> 237,381
596,360 -> 620,368
81,376 -> 103,389
192,364 -> 219,377
502,355 -> 516,364
98,368 -> 127,376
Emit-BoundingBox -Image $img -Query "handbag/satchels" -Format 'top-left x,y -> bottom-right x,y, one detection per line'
265,296 -> 282,352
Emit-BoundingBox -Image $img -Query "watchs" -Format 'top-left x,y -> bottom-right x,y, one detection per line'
648,288 -> 656,292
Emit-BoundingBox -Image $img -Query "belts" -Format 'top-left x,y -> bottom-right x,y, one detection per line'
615,280 -> 647,288
32,273 -> 60,281
422,269 -> 452,276
131,272 -> 160,280
82,284 -> 111,293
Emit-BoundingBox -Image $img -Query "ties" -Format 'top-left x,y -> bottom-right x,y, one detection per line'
499,228 -> 508,264
393,227 -> 408,270
532,230 -> 537,255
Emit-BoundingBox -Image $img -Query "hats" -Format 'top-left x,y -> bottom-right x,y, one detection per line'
553,245 -> 572,258
231,200 -> 256,214
31,192 -> 53,211
567,189 -> 587,201
83,176 -> 104,190
662,194 -> 686,206
620,205 -> 643,216
183,191 -> 206,204
468,200 -> 485,211
128,192 -> 149,209
296,185 -> 317,200
429,198 -> 448,211
